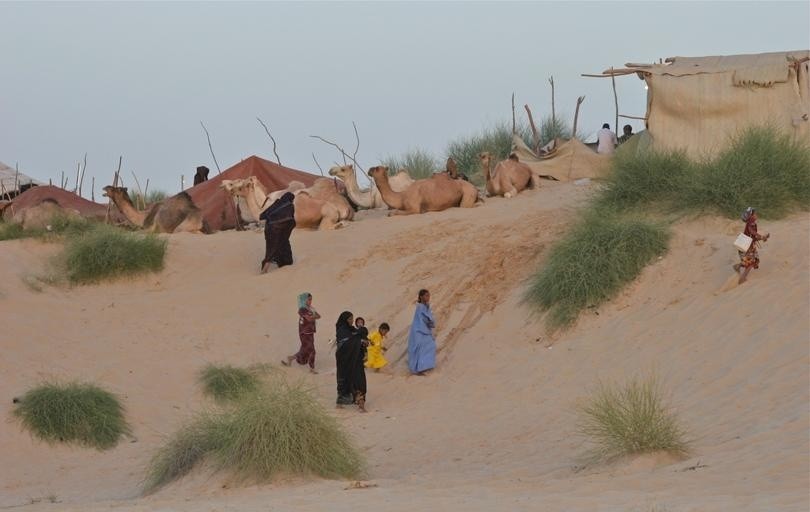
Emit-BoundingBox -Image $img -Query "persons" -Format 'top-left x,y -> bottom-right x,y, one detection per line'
732,206 -> 768,286
596,124 -> 618,156
408,289 -> 436,376
355,317 -> 370,363
280,292 -> 321,374
193,166 -> 209,186
618,125 -> 634,147
335,311 -> 370,413
363,322 -> 390,373
257,191 -> 296,276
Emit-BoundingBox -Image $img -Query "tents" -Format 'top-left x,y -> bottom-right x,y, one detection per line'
4,184 -> 117,228
152,153 -> 345,232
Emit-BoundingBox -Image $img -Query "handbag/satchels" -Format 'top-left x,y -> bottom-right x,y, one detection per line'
735,233 -> 753,252
